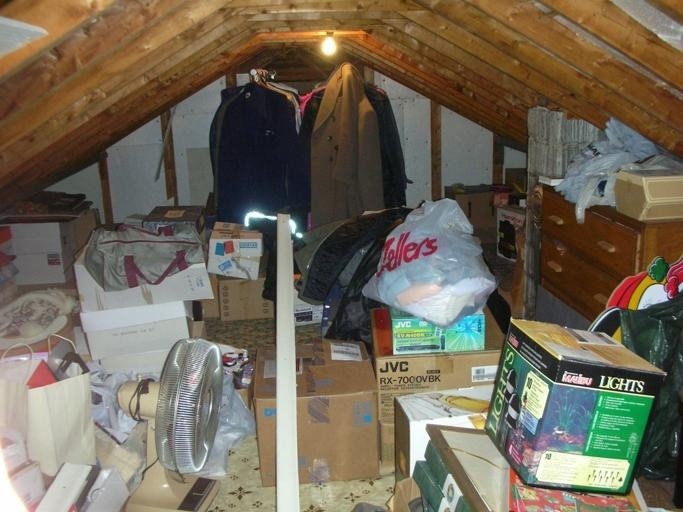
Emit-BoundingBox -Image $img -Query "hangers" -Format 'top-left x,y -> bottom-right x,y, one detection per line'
244,69 -> 270,90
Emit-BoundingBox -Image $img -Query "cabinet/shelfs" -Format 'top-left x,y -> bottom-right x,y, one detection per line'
537,180 -> 682,323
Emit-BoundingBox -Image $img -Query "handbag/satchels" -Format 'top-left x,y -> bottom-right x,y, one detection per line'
85,222 -> 206,292
2,334 -> 96,476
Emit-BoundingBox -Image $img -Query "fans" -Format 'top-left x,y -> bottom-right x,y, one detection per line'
116,337 -> 224,512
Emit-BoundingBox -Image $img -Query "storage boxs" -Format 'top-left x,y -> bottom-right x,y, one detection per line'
485,318 -> 666,493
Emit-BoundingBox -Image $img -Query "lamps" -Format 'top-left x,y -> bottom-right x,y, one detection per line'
320,32 -> 337,57
